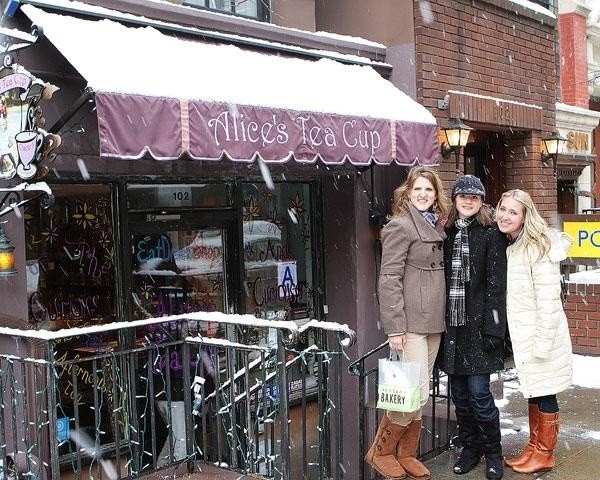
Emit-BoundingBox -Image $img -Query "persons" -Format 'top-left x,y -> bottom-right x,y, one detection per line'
447,174 -> 505,478
495,187 -> 573,473
200,321 -> 221,376
366,165 -> 447,478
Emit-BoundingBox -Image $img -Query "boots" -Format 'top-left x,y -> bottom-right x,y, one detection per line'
364,415 -> 407,480
474,407 -> 503,480
396,420 -> 431,480
505,404 -> 538,467
453,410 -> 484,474
512,409 -> 560,474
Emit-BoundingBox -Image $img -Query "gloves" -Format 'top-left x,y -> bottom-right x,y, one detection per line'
484,336 -> 501,351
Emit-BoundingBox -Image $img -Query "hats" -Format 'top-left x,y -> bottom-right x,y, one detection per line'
452,175 -> 486,202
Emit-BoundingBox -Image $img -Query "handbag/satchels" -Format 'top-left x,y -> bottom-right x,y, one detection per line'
376,350 -> 421,413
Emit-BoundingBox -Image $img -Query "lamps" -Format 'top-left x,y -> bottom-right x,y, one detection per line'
0,225 -> 18,279
435,116 -> 472,170
539,125 -> 570,175
